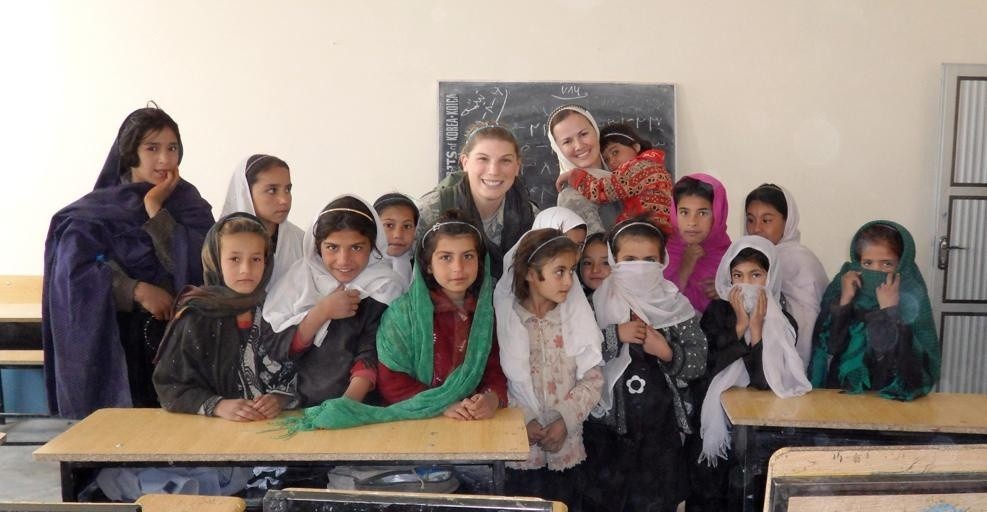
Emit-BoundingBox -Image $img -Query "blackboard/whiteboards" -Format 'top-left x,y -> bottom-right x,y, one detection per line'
438,81 -> 675,211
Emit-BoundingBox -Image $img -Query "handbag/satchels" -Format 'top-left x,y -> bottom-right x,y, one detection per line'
327,466 -> 460,494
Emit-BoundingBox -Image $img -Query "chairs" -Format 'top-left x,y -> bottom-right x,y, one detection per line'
262,486 -> 569,512
1,495 -> 248,511
765,441 -> 987,512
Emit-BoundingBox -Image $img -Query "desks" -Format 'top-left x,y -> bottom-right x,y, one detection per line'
2,273 -> 44,430
719,386 -> 987,512
32,404 -> 530,503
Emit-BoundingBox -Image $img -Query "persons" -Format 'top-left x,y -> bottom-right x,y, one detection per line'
151,101 -> 830,510
42,99 -> 215,502
807,219 -> 942,401
